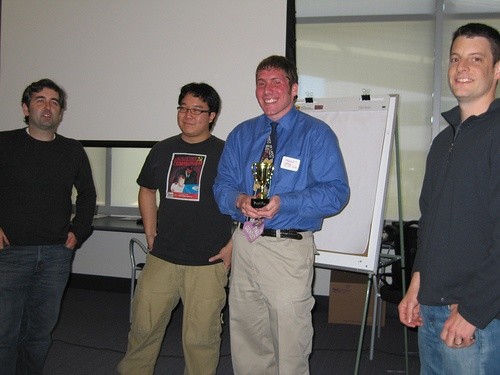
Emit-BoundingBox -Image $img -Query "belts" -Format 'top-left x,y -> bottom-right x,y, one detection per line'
240,222 -> 309,240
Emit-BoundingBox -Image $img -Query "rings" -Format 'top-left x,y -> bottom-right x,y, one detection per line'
454,342 -> 462,346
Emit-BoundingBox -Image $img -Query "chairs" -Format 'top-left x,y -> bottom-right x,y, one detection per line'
129,238 -> 149,324
370,226 -> 418,359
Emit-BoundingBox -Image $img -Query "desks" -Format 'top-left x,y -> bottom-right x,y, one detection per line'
69,212 -> 145,233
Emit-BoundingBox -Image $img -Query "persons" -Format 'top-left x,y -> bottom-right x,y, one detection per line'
116,82 -> 234,375
210,53 -> 350,375
0,78 -> 99,375
398,24 -> 500,375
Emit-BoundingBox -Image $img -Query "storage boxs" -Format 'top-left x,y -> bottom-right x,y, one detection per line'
327,267 -> 387,326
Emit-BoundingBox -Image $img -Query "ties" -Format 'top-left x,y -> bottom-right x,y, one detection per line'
252,120 -> 280,200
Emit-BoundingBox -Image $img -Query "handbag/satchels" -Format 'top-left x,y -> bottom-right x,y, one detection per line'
378,219 -> 418,304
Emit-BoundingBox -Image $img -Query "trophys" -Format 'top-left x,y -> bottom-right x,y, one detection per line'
250,163 -> 275,208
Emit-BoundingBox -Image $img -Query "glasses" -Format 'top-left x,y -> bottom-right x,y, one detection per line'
176,106 -> 209,115
